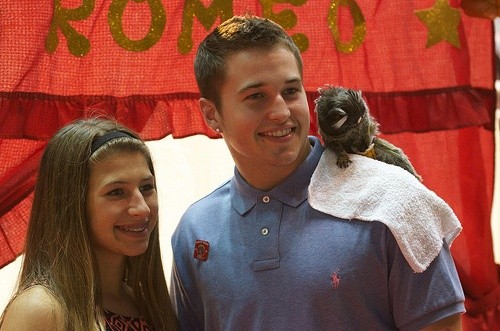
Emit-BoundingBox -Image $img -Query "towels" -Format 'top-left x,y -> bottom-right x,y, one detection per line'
307,148 -> 463,274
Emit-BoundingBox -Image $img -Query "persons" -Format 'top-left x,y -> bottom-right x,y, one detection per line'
0,115 -> 180,331
170,15 -> 466,331
